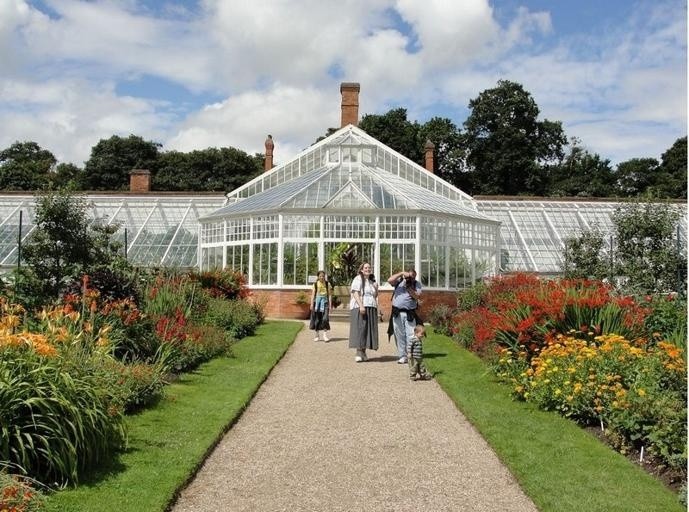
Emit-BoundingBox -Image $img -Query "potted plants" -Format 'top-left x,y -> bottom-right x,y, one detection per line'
331,242 -> 361,309
289,291 -> 311,319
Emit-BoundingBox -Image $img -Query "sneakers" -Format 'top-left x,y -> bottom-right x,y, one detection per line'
313,332 -> 330,344
353,350 -> 432,382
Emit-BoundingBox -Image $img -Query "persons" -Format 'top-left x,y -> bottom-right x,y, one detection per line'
387,268 -> 422,363
349,262 -> 379,363
309,270 -> 333,343
405,324 -> 432,381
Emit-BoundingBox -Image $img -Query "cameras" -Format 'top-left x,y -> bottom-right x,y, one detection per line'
405,277 -> 417,288
